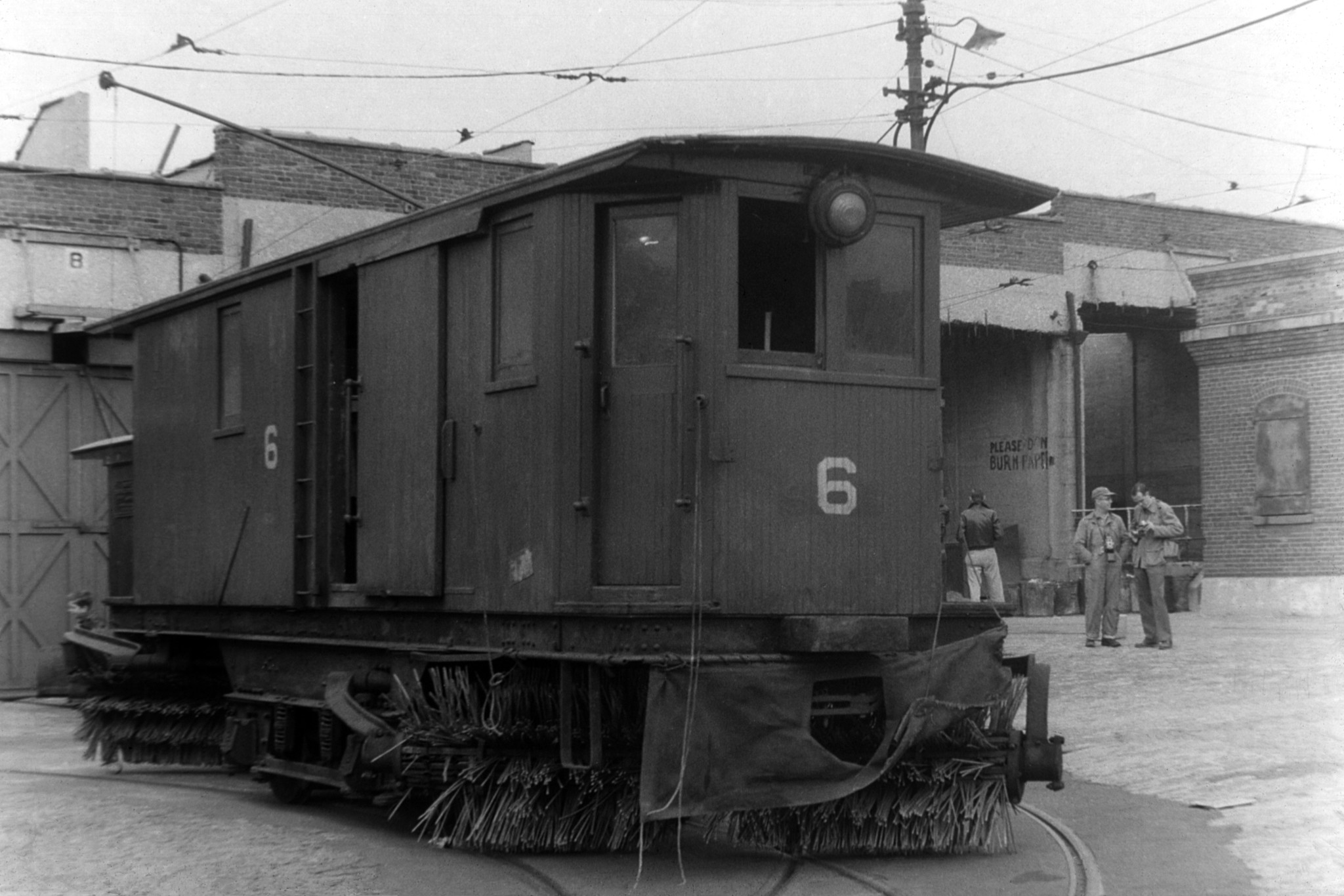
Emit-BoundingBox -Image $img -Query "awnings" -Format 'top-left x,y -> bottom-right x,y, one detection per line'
1062,241 -> 1198,316
939,264 -> 1084,337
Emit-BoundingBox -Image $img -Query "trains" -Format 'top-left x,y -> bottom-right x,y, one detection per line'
63,135 -> 1065,851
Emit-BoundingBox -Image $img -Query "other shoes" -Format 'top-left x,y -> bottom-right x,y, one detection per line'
1135,640 -> 1156,648
1158,640 -> 1171,649
1101,637 -> 1120,647
1085,639 -> 1095,647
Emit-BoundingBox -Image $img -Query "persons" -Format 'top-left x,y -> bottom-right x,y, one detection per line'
67,591 -> 100,630
941,490 -> 950,601
1073,486 -> 1132,647
1124,481 -> 1184,650
959,488 -> 1014,617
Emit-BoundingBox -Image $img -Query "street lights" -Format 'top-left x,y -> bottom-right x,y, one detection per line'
882,0 -> 1005,154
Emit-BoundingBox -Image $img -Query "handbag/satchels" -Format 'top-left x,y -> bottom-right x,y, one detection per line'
1164,541 -> 1179,557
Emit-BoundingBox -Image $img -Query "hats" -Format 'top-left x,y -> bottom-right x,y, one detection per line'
1092,486 -> 1116,498
